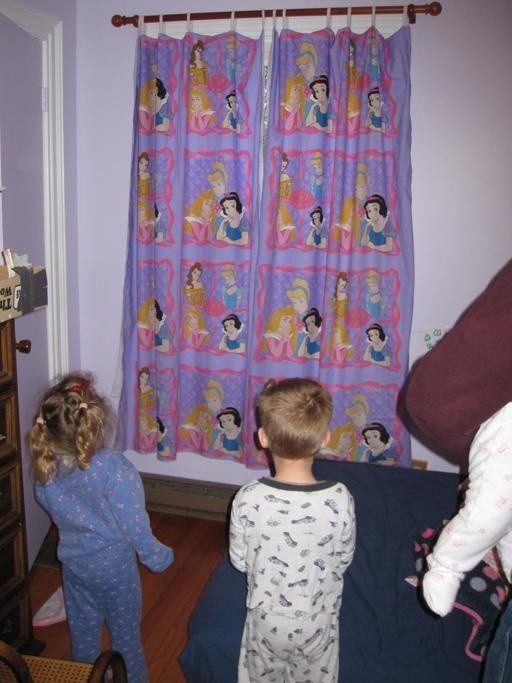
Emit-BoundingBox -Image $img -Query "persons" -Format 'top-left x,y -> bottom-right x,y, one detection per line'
224,374 -> 362,683
397,254 -> 512,619
20,368 -> 177,682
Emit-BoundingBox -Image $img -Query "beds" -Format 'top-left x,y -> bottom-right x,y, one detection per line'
178,455 -> 511,681
